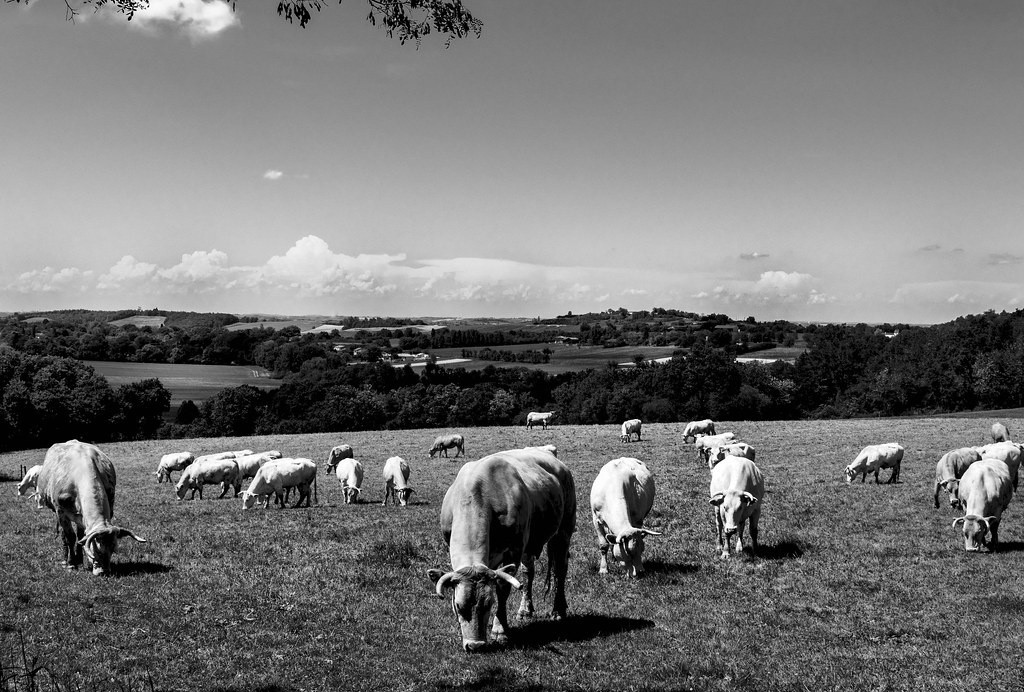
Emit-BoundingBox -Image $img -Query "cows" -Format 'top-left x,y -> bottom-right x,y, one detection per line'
589,455 -> 662,580
844,442 -> 905,485
151,451 -> 195,484
708,454 -> 765,559
236,456 -> 320,510
424,444 -> 579,655
692,431 -> 735,464
336,457 -> 365,504
174,448 -> 283,501
526,411 -> 558,430
36,438 -> 148,576
681,418 -> 717,444
990,422 -> 1010,443
321,444 -> 354,475
707,442 -> 756,471
381,455 -> 418,507
933,440 -> 1024,552
619,418 -> 642,443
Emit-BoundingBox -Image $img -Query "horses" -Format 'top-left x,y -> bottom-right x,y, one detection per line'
13,464 -> 43,496
